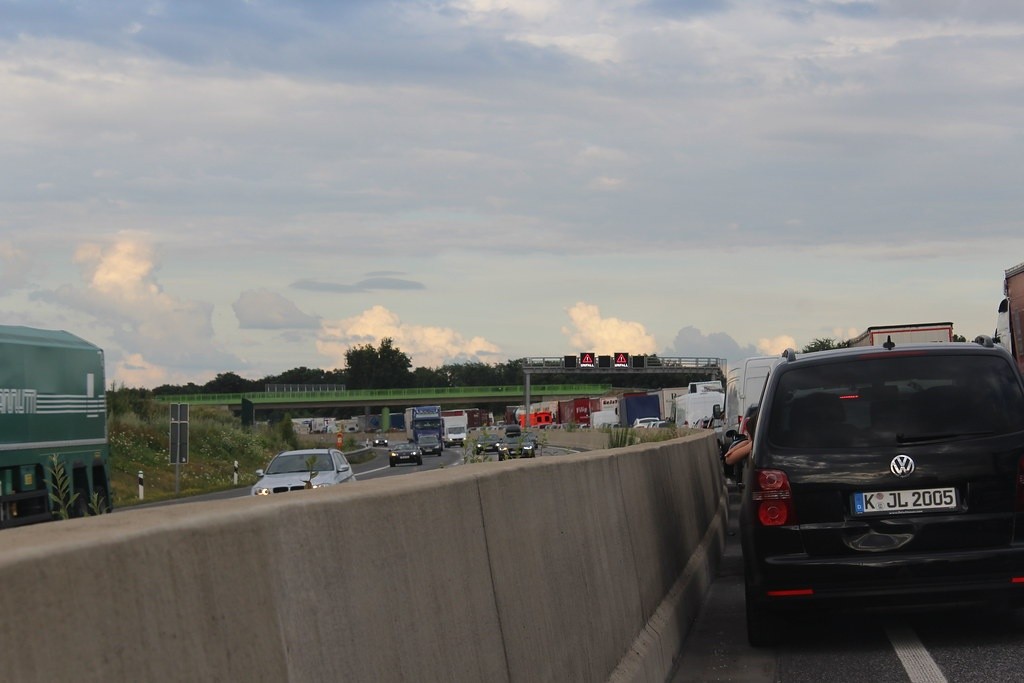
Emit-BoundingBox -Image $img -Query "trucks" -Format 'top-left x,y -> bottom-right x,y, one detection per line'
504,380 -> 727,430
440,409 -> 468,448
405,405 -> 444,445
846,321 -> 954,348
992,262 -> 1024,380
0,324 -> 115,530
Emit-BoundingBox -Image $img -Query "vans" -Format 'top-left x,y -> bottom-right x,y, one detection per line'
712,354 -> 782,479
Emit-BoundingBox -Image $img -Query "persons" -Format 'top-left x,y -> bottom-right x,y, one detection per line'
724,413 -> 757,465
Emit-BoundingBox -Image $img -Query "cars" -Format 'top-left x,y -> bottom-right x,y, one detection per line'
475,433 -> 503,455
389,443 -> 422,467
523,432 -> 539,449
373,436 -> 388,447
497,436 -> 535,461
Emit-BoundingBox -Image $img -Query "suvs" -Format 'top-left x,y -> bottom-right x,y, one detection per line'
250,448 -> 357,497
418,435 -> 442,457
725,335 -> 1024,650
